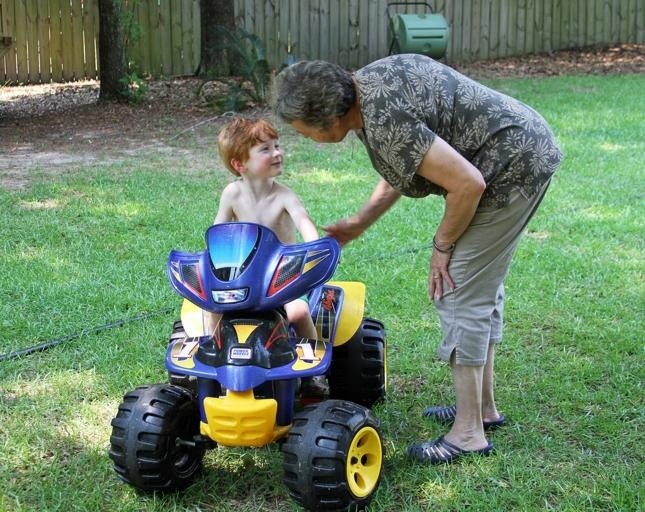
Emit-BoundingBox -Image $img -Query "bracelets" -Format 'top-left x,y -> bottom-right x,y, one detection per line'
433,234 -> 456,254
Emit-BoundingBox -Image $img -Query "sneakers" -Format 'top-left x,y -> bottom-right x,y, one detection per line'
307,375 -> 330,397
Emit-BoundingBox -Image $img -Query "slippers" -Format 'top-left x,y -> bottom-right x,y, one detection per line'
424,403 -> 505,429
405,433 -> 495,465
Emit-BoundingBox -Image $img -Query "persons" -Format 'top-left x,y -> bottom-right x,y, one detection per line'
267,54 -> 565,465
198,114 -> 324,341
